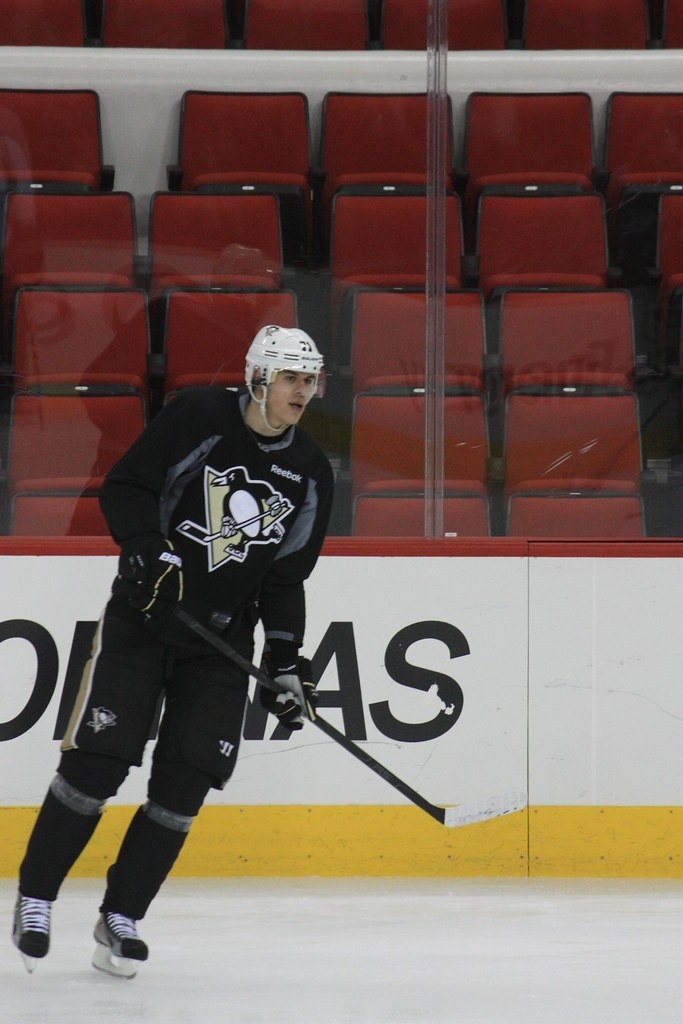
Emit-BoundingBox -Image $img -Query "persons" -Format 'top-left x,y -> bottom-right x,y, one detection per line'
10,323 -> 334,979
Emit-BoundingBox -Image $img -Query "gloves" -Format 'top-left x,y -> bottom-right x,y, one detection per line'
259,642 -> 319,732
124,537 -> 182,629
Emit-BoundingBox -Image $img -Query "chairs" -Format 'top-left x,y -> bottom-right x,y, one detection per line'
0,0 -> 683,50
0,89 -> 683,537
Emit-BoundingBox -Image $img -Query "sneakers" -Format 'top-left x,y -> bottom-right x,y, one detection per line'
92,907 -> 147,979
11,892 -> 54,972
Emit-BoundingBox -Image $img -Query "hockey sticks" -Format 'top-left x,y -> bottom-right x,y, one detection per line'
154,585 -> 532,832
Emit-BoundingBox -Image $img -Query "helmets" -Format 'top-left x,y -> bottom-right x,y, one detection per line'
245,327 -> 322,401
